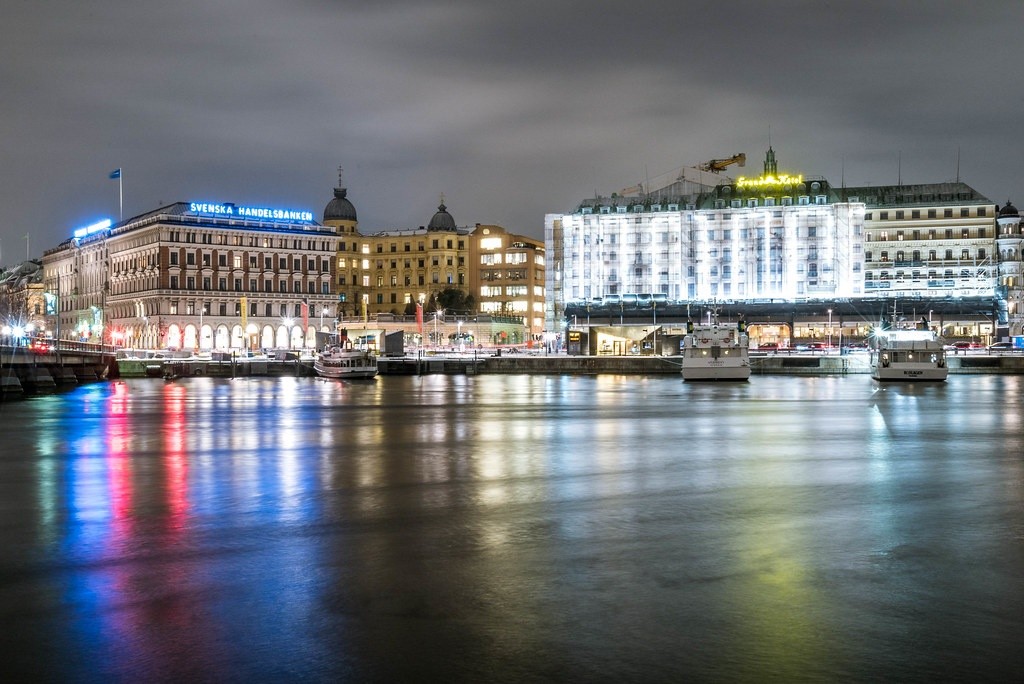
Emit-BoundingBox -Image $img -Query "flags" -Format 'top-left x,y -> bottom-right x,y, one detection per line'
416,303 -> 423,333
110,169 -> 120,178
94,309 -> 101,325
240,297 -> 247,331
47,295 -> 56,315
301,301 -> 308,332
361,299 -> 368,322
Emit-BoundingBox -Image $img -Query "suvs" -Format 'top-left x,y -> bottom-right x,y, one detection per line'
986,341 -> 1013,352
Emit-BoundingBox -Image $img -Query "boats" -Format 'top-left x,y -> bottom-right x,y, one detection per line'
871,299 -> 949,381
313,339 -> 379,380
681,297 -> 752,381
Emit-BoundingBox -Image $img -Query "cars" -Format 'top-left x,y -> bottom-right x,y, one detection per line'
757,342 -> 779,352
951,340 -> 971,349
